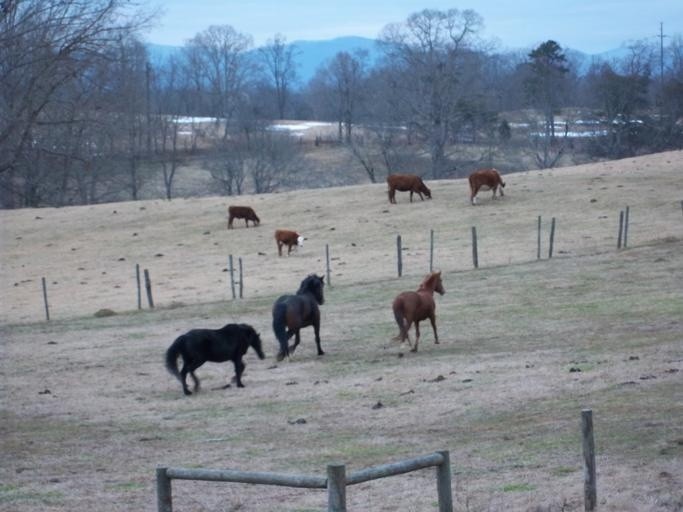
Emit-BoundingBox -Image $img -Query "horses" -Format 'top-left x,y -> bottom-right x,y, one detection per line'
164,323 -> 265,395
272,273 -> 325,361
392,270 -> 445,351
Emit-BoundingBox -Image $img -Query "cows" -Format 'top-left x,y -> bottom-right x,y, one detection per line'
227,206 -> 260,230
274,229 -> 308,257
387,174 -> 432,204
469,168 -> 506,205
